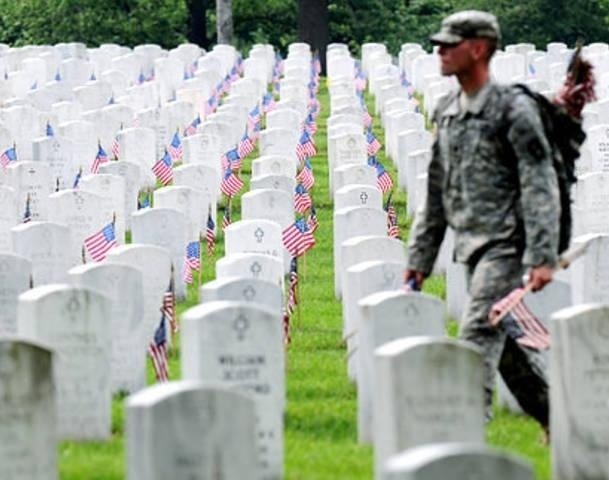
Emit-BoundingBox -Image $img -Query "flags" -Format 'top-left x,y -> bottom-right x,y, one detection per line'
353,56 -> 421,238
489,287 -> 553,353
223,51 -> 322,254
150,243 -> 304,382
2,56 -> 243,259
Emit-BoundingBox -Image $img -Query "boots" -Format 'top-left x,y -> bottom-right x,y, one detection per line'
539,425 -> 550,447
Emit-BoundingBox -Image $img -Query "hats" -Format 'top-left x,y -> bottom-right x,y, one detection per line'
430,10 -> 501,47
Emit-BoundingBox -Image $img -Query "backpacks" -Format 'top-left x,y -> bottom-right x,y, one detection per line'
512,83 -> 586,258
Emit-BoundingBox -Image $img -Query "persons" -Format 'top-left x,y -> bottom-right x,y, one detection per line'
401,9 -> 563,446
